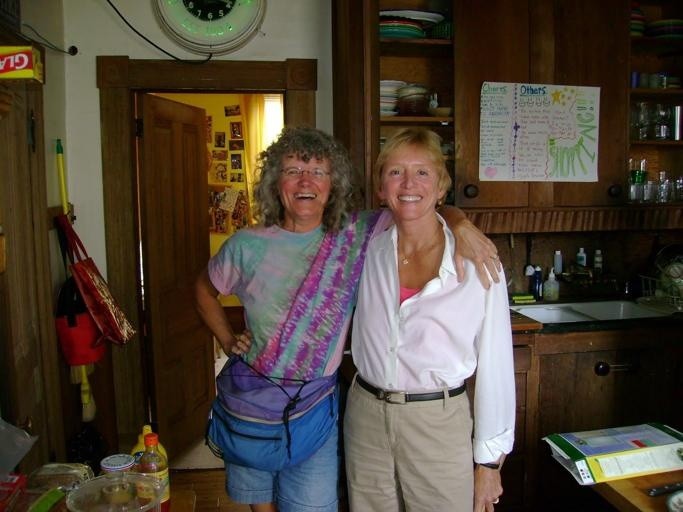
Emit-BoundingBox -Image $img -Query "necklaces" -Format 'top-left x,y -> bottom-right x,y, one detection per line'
397,223 -> 442,265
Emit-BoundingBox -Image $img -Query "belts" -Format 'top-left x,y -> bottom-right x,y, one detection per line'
356,375 -> 466,405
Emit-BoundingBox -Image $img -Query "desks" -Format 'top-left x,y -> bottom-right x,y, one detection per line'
591,429 -> 683,512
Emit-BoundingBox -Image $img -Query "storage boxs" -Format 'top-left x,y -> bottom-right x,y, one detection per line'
0,41 -> 46,84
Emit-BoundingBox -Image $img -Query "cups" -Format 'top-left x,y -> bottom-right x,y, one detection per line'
632,101 -> 672,141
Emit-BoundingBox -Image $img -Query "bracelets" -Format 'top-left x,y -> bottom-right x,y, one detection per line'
477,463 -> 500,469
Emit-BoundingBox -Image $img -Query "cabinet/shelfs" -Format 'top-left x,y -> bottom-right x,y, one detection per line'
0,79 -> 68,479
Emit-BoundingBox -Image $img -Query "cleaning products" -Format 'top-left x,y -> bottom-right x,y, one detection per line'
553,250 -> 564,273
593,249 -> 604,270
576,247 -> 587,266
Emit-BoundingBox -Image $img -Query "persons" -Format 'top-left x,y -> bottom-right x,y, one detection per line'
343,126 -> 516,511
190,122 -> 501,512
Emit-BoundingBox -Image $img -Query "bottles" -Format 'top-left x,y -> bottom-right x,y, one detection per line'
554,247 -> 603,275
100,425 -> 170,512
628,158 -> 683,203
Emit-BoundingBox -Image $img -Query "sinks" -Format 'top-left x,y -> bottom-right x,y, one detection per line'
571,300 -> 665,324
509,304 -> 592,323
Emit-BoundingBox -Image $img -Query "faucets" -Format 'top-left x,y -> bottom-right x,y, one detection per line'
622,276 -> 632,299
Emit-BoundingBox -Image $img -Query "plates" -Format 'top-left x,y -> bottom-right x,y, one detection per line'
380,80 -> 451,117
664,490 -> 683,512
380,10 -> 444,38
629,13 -> 682,39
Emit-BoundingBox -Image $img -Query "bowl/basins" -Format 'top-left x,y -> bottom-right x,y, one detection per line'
660,263 -> 682,294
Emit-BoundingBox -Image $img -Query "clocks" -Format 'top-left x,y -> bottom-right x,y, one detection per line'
151,0 -> 267,56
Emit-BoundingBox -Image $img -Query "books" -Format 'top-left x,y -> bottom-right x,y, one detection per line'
0,472 -> 29,512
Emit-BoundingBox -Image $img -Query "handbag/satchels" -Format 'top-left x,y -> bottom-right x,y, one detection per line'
205,355 -> 340,472
54,253 -> 137,365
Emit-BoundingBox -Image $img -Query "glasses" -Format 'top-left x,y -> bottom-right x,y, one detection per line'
281,168 -> 331,178
